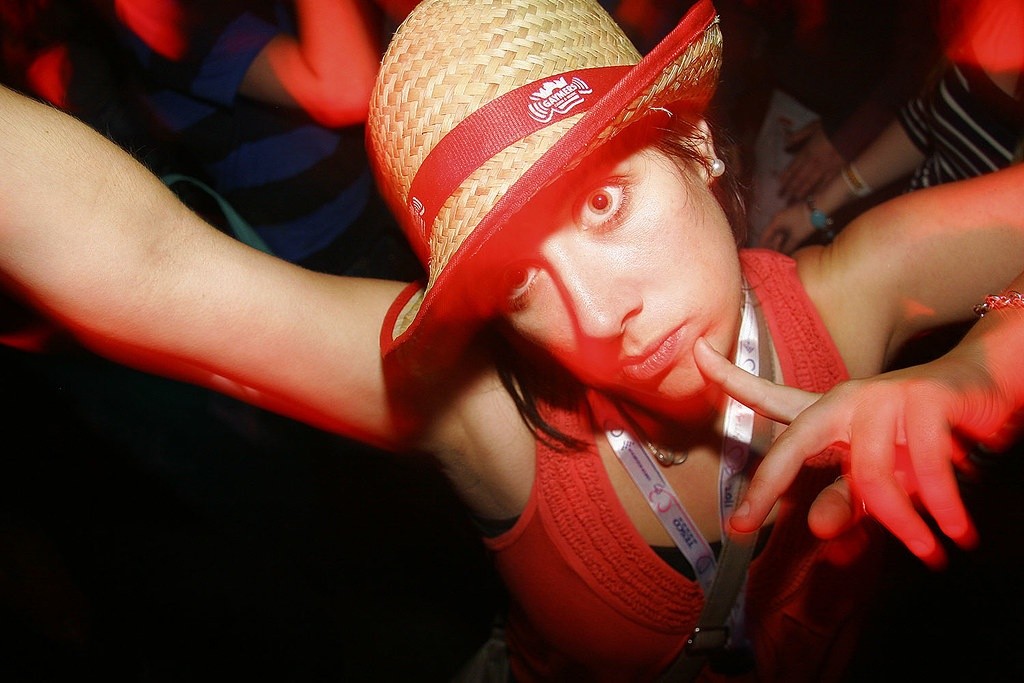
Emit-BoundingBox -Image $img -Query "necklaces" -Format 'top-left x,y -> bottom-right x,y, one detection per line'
617,397 -> 696,469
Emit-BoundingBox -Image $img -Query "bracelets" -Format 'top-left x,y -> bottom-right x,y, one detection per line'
806,193 -> 836,230
840,157 -> 872,199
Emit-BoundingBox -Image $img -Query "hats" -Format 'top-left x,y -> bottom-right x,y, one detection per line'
363,0 -> 727,362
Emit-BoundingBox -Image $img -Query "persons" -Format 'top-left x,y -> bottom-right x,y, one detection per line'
1,0 -> 1024,683
742,2 -> 935,250
753,5 -> 1024,257
